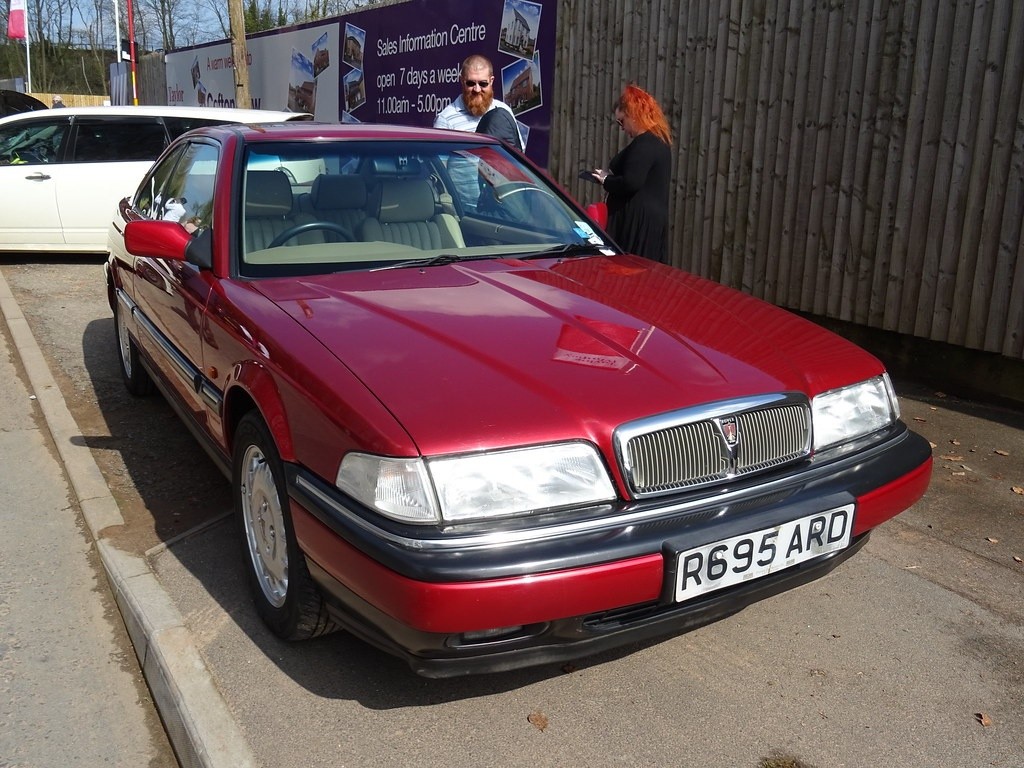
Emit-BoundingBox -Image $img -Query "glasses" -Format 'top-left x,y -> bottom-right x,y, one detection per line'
615,113 -> 626,126
462,75 -> 489,87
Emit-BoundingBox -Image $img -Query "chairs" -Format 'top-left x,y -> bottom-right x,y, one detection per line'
245,167 -> 325,252
359,178 -> 466,247
307,171 -> 376,240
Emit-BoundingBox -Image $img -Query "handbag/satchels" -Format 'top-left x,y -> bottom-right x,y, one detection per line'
588,202 -> 608,231
474,107 -> 523,154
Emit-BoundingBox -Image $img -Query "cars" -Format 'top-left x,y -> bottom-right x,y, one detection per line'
0,105 -> 329,264
103,122 -> 933,685
0,90 -> 49,115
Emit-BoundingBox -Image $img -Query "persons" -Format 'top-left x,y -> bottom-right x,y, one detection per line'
592,85 -> 671,266
155,192 -> 162,205
52,96 -> 66,108
433,54 -> 558,231
162,198 -> 187,223
182,216 -> 210,238
